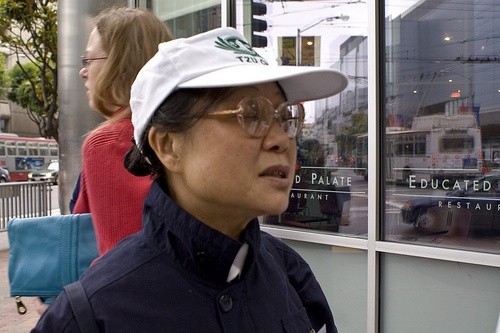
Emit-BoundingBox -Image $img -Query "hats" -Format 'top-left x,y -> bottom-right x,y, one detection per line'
129,27 -> 350,154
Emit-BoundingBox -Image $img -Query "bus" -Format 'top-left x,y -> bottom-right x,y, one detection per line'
353,71 -> 484,188
0,133 -> 60,182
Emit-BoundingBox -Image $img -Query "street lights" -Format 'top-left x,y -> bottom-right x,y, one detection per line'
295,14 -> 350,66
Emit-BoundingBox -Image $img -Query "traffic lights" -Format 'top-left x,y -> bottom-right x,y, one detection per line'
251,1 -> 269,50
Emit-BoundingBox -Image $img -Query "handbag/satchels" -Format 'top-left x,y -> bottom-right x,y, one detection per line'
4,213 -> 101,298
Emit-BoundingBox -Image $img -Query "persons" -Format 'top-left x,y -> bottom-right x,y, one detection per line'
282,138 -> 338,233
72,2 -> 174,269
482,164 -> 488,175
494,152 -> 500,162
30,27 -> 349,333
487,160 -> 492,171
69,172 -> 82,214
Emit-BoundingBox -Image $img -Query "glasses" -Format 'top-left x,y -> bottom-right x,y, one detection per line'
180,95 -> 305,138
79,53 -> 112,67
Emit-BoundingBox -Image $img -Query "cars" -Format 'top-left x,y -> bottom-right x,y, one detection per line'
400,172 -> 500,237
27,158 -> 61,185
